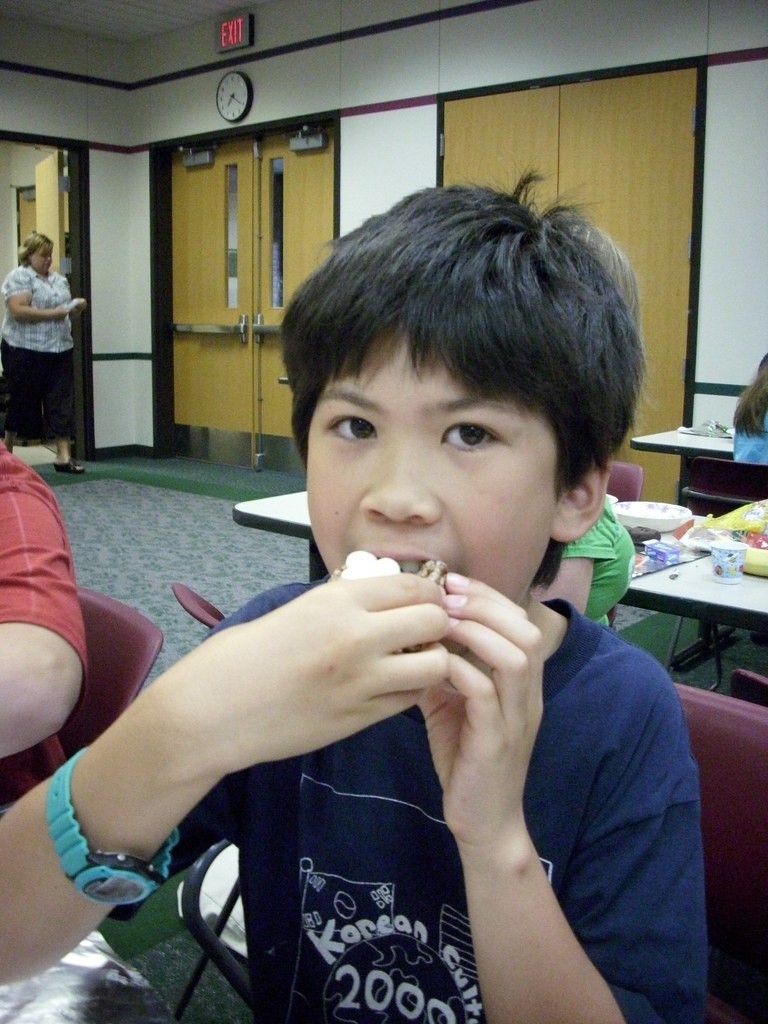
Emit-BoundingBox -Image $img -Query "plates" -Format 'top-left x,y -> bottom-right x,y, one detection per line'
606,493 -> 618,506
611,501 -> 692,532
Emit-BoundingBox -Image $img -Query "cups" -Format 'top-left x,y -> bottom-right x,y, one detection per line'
711,539 -> 748,585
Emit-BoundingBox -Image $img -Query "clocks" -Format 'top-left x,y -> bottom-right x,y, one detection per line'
216,70 -> 255,122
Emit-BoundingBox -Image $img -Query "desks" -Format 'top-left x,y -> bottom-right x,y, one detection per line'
233,491 -> 768,692
631,431 -> 736,670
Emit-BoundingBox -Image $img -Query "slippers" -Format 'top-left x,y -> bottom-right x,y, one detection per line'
53,458 -> 85,474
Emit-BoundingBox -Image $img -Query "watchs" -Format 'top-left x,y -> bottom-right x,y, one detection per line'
46,747 -> 178,905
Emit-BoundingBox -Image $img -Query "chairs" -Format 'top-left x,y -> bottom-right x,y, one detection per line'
67,462 -> 767,1024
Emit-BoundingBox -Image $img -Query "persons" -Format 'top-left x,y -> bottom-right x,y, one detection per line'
732,353 -> 768,465
532,493 -> 636,628
0,363 -> 89,808
0,231 -> 88,474
0,169 -> 707,1024
